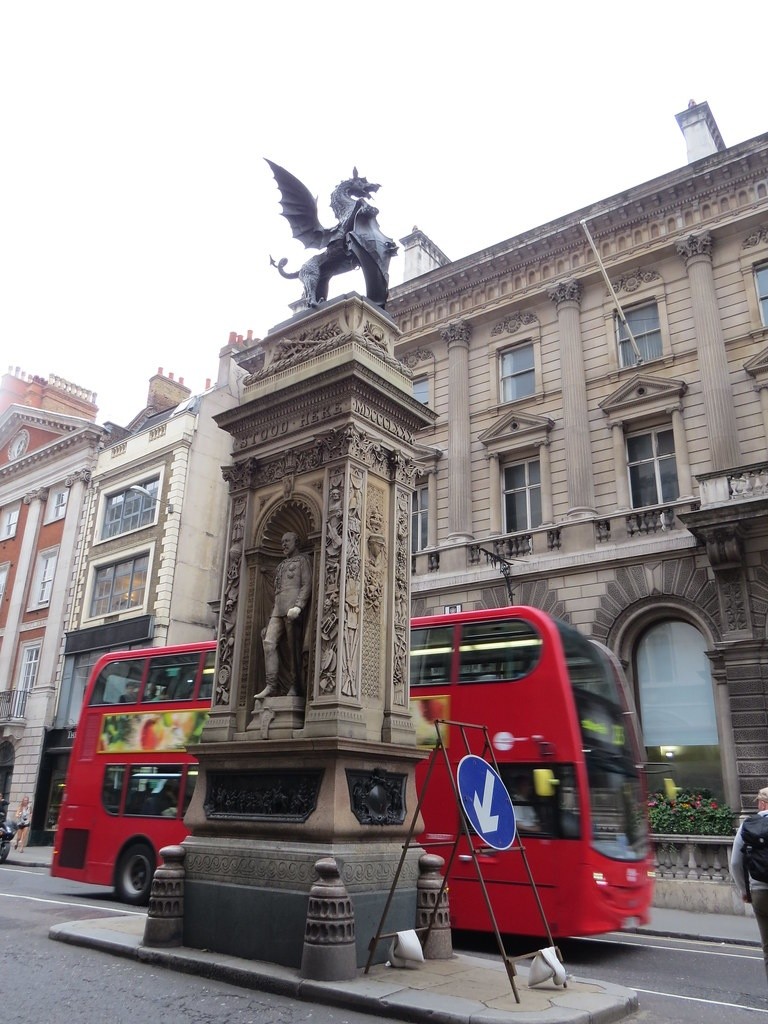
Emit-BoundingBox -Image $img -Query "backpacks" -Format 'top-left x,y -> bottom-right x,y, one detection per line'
740,813 -> 768,883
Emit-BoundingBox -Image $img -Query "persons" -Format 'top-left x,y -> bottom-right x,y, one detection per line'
503,775 -> 542,836
730,786 -> 767,985
105,650 -> 221,717
14,795 -> 33,853
107,763 -> 197,822
0,793 -> 10,823
252,531 -> 313,699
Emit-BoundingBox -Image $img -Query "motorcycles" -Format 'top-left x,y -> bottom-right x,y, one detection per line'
0,812 -> 18,864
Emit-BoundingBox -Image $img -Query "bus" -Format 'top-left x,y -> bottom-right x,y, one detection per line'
51,605 -> 678,938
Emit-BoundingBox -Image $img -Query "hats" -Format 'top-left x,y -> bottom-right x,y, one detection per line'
752,787 -> 768,803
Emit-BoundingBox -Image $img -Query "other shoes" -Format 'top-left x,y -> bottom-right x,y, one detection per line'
287,681 -> 303,696
254,672 -> 279,699
14,845 -> 18,850
20,849 -> 23,853
